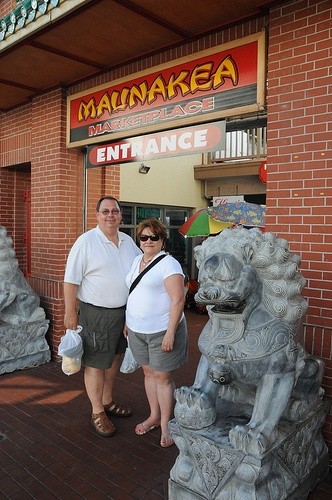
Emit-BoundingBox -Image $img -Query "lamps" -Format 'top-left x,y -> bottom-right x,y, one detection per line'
139,163 -> 151,174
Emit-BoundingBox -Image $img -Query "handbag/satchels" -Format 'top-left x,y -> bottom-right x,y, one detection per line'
58,325 -> 84,376
119,336 -> 141,373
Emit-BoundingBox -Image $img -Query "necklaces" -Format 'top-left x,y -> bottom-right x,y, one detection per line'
141,258 -> 149,265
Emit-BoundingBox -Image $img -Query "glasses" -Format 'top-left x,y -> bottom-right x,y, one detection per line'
98,209 -> 121,215
140,234 -> 162,242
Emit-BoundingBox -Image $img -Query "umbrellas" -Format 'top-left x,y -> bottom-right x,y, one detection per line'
178,202 -> 266,238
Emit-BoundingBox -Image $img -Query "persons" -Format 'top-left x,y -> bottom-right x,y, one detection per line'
64,197 -> 143,436
122,218 -> 189,448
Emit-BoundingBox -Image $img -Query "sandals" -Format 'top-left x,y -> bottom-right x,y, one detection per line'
91,410 -> 116,436
103,400 -> 133,417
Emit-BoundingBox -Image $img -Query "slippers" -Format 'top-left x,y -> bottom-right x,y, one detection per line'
160,434 -> 175,447
135,421 -> 159,435
